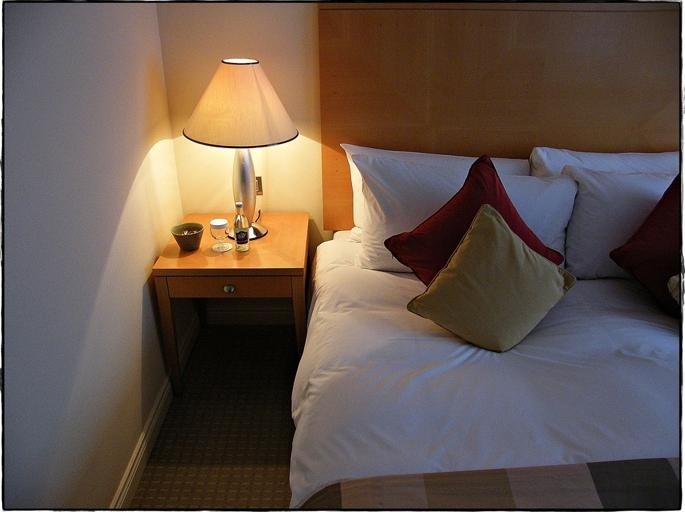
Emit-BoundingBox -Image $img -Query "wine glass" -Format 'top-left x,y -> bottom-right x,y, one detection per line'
209,219 -> 233,253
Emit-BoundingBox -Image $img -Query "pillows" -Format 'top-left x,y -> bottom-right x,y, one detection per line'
339,142 -> 531,244
610,173 -> 680,324
350,154 -> 579,273
530,146 -> 680,177
560,162 -> 680,279
404,203 -> 578,354
384,155 -> 569,286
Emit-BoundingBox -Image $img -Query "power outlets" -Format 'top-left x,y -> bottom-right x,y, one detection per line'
256,177 -> 263,195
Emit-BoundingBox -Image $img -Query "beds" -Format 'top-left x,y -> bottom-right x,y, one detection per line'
288,229 -> 681,511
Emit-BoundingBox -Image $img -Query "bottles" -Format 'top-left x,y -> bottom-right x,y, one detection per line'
232,202 -> 249,251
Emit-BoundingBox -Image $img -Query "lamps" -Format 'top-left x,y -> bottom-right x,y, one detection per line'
182,59 -> 299,241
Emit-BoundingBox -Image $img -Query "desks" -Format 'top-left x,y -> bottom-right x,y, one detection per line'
152,211 -> 309,398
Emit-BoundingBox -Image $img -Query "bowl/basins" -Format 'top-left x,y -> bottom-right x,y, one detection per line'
170,222 -> 204,251
